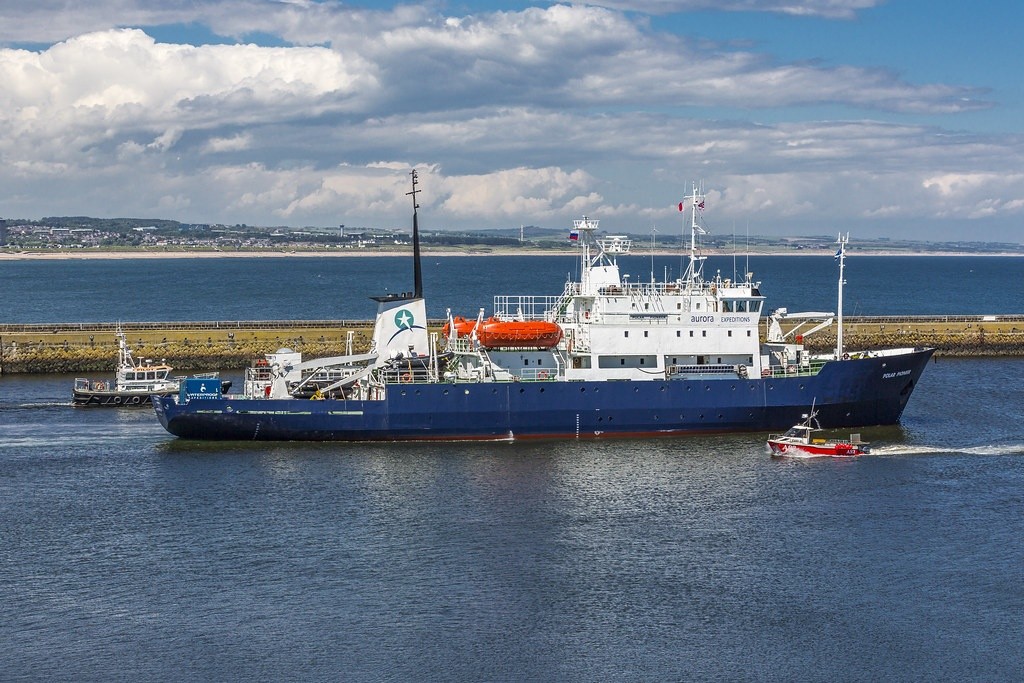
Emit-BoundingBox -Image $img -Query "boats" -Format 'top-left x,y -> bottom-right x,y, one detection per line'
764,396 -> 871,458
71,317 -> 233,407
152,163 -> 942,443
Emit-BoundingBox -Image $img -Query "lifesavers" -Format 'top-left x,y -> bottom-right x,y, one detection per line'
401,374 -> 411,383
538,371 -> 547,380
844,354 -> 849,359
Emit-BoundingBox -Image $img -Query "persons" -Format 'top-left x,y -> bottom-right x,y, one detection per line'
841,351 -> 850,360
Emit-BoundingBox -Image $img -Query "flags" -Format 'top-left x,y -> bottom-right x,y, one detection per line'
699,201 -> 704,209
678,203 -> 683,211
